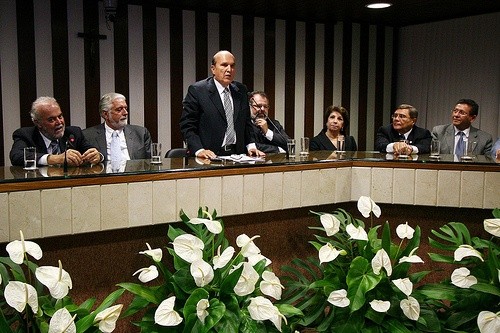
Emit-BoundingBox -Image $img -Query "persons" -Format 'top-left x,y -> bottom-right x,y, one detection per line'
491,137 -> 500,158
9,96 -> 105,166
430,99 -> 492,154
249,91 -> 292,153
83,93 -> 153,161
376,104 -> 432,154
309,105 -> 357,150
181,50 -> 266,158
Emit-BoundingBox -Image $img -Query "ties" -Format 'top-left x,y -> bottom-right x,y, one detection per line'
455,132 -> 466,153
51,140 -> 60,155
222,87 -> 236,146
400,134 -> 405,140
111,131 -> 122,173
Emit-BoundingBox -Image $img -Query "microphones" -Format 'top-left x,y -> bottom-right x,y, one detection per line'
63,126 -> 69,173
246,91 -> 290,161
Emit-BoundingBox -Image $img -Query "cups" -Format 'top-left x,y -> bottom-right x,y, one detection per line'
24,147 -> 37,170
461,142 -> 472,160
496,141 -> 500,160
336,136 -> 345,152
286,139 -> 295,157
150,143 -> 161,163
431,139 -> 441,159
398,140 -> 409,160
300,137 -> 309,154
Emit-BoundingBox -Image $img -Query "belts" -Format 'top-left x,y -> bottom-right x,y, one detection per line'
219,144 -> 236,151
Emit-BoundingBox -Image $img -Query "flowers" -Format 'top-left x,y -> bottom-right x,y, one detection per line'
115,206 -> 305,333
278,195 -> 444,333
0,230 -> 126,333
415,207 -> 500,333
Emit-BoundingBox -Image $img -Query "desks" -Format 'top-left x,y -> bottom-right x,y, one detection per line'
0,149 -> 500,245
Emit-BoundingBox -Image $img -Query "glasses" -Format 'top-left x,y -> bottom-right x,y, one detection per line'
392,114 -> 410,119
253,104 -> 270,110
451,108 -> 470,115
